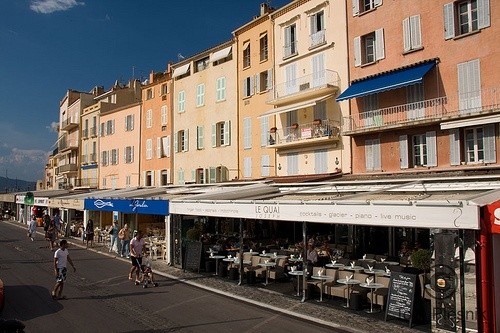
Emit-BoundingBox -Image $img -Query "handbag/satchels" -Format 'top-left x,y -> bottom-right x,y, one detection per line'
26,232 -> 31,237
109,225 -> 113,234
118,228 -> 125,239
45,232 -> 50,238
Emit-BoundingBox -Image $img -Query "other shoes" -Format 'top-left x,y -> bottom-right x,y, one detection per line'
51,289 -> 56,297
57,295 -> 64,299
120,254 -> 123,257
135,279 -> 140,283
126,256 -> 130,258
128,273 -> 132,279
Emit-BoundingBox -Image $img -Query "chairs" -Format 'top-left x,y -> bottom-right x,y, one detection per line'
227,244 -> 353,283
306,254 -> 432,312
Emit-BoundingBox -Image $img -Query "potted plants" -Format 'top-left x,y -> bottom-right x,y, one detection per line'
403,249 -> 432,326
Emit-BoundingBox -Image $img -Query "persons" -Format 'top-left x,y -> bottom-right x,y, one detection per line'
308,238 -> 317,265
44,213 -> 64,250
49,240 -> 76,299
117,224 -> 132,259
29,218 -> 36,241
106,219 -> 121,253
86,219 -> 95,245
129,232 -> 148,283
141,265 -> 154,286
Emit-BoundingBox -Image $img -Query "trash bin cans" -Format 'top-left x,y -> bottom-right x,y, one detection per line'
229,267 -> 238,278
218,264 -> 228,276
350,292 -> 361,310
304,283 -> 314,299
246,270 -> 257,283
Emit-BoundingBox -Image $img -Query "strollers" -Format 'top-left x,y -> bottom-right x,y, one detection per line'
136,256 -> 157,288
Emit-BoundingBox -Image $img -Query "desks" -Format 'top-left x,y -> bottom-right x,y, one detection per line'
259,253 -> 271,257
364,269 -> 385,275
257,261 -> 278,288
234,259 -> 251,281
337,279 -> 360,308
344,266 -> 364,271
209,256 -> 225,276
310,273 -> 334,303
359,283 -> 383,314
325,264 -> 344,268
288,270 -> 310,297
226,248 -> 240,256
271,254 -> 288,264
221,257 -> 237,279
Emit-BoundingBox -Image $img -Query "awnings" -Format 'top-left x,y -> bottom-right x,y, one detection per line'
256,92 -> 334,121
49,135 -> 65,149
336,57 -> 437,102
172,63 -> 191,78
209,46 -> 232,63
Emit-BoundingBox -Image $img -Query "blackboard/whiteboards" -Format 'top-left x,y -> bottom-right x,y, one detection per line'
181,240 -> 202,271
385,271 -> 416,324
